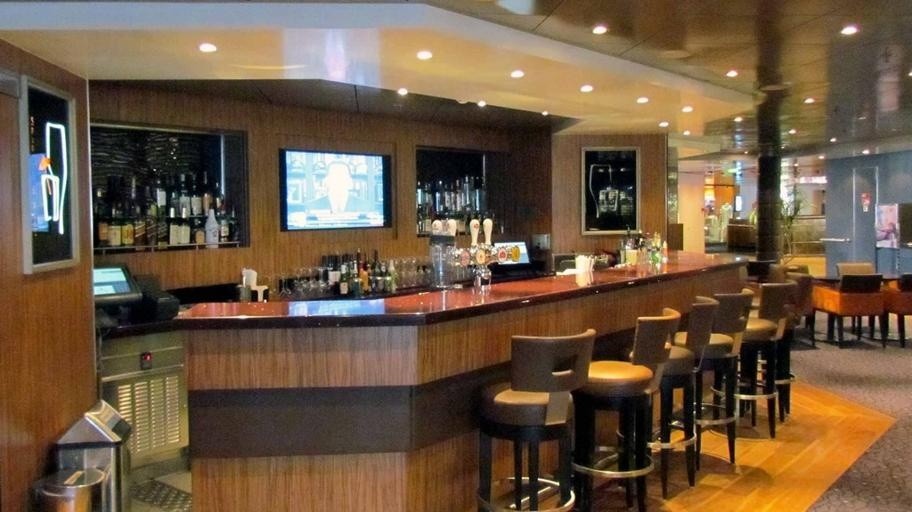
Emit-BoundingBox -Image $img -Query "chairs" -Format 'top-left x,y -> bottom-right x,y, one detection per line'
770,261 -> 912,349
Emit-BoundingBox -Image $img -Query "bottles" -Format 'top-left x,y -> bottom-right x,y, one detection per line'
320,247 -> 397,295
416,175 -> 496,236
622,223 -> 668,265
472,264 -> 492,295
92,168 -> 238,251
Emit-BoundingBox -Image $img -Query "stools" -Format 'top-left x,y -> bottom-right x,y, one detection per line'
630,295 -> 719,499
471,326 -> 598,511
761,273 -> 813,414
666,289 -> 756,472
573,306 -> 681,512
711,277 -> 799,439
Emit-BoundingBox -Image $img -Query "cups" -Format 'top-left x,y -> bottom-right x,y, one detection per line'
429,238 -> 456,290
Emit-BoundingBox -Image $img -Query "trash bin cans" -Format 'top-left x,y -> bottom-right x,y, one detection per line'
52,399 -> 134,512
43,468 -> 108,512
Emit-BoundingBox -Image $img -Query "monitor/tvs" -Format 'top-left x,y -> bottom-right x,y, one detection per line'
278,147 -> 392,232
93,263 -> 143,327
493,238 -> 532,265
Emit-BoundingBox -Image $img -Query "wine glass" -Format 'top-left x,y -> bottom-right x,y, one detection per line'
398,255 -> 430,288
268,266 -> 328,302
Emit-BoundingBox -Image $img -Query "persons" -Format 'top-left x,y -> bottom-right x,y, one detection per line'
878,205 -> 897,230
719,202 -> 733,243
706,200 -> 715,216
